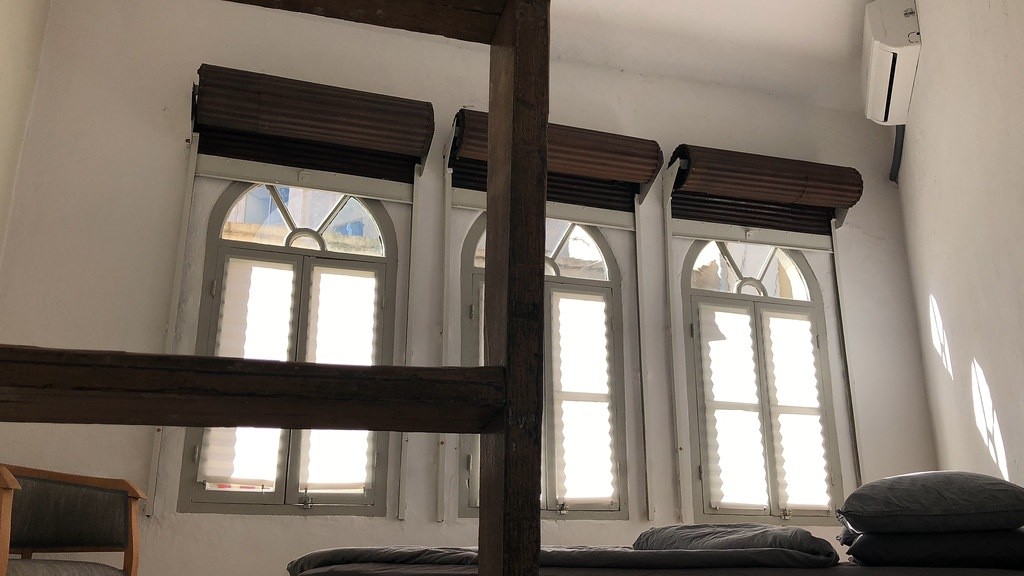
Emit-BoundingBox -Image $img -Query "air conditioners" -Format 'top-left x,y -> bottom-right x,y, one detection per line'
860,0 -> 921,126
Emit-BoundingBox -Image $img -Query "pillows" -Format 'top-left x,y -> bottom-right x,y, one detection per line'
845,530 -> 1024,570
835,526 -> 855,546
834,469 -> 1024,536
632,522 -> 841,561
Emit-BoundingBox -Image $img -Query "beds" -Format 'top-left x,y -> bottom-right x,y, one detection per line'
205,548 -> 1024,576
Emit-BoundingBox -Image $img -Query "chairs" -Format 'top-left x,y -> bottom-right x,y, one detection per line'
0,463 -> 147,576
